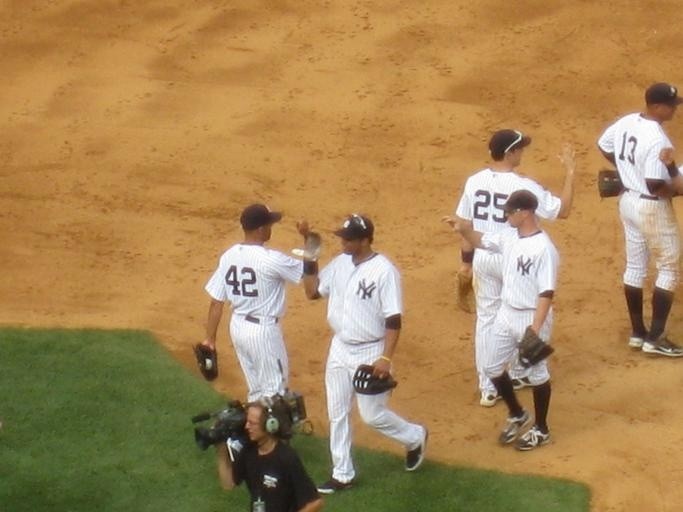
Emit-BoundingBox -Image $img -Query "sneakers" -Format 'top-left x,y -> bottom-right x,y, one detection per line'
406,425 -> 428,471
317,478 -> 353,494
513,424 -> 551,451
498,410 -> 531,444
642,332 -> 683,357
628,336 -> 643,348
512,377 -> 535,390
479,389 -> 504,407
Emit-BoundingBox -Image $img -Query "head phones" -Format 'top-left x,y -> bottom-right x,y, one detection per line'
257,395 -> 279,433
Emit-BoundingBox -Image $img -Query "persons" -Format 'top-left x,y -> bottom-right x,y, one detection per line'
440,190 -> 559,451
659,147 -> 683,195
212,399 -> 323,512
303,214 -> 428,494
595,82 -> 683,356
455,129 -> 578,408
196,204 -> 320,408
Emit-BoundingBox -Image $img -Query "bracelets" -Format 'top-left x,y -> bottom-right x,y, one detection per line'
379,356 -> 393,364
303,261 -> 319,275
664,159 -> 679,177
460,247 -> 475,263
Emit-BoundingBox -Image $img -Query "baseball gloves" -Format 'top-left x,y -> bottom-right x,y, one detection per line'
599,169 -> 622,198
353,363 -> 396,394
193,342 -> 219,380
456,271 -> 479,314
517,327 -> 555,368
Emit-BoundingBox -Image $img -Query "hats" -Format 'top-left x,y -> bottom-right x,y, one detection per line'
489,129 -> 531,153
333,212 -> 374,240
240,204 -> 282,229
645,82 -> 683,105
496,189 -> 538,212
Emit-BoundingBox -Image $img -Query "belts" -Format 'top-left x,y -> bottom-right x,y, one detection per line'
246,316 -> 278,324
624,188 -> 658,200
